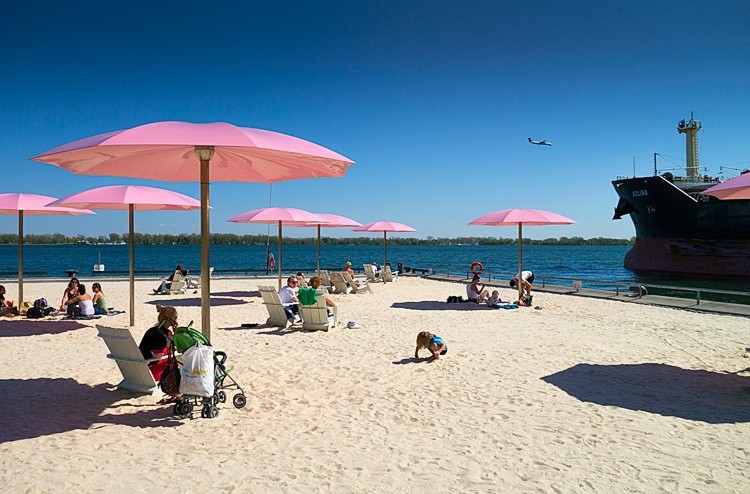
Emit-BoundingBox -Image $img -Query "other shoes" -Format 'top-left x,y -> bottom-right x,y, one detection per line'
348,320 -> 360,329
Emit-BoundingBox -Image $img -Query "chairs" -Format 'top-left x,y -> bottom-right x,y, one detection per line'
96,324 -> 182,394
170,274 -> 185,295
187,267 -> 214,287
300,307 -> 336,331
257,285 -> 301,331
297,264 -> 398,296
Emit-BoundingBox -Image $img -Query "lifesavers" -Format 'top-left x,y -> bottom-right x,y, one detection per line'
470,262 -> 483,276
269,257 -> 275,268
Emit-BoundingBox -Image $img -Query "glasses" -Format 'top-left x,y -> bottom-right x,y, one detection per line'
289,281 -> 299,284
71,281 -> 78,283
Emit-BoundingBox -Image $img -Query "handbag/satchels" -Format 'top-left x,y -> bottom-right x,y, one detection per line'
26,297 -> 56,318
179,339 -> 215,399
158,340 -> 181,396
519,293 -> 533,306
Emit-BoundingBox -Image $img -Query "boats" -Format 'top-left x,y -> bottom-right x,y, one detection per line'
610,111 -> 750,284
77,239 -> 127,246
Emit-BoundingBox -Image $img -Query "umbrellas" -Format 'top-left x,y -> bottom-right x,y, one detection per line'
277,213 -> 364,278
352,221 -> 418,272
699,172 -> 750,200
227,207 -> 329,292
44,185 -> 214,326
0,193 -> 96,301
28,121 -> 355,344
467,209 -> 578,295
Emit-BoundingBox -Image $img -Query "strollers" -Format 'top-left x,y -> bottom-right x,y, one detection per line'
167,318 -> 246,419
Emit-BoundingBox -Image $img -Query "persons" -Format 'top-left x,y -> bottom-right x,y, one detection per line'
0,285 -> 17,317
510,270 -> 534,297
371,262 -> 381,281
61,278 -> 109,317
467,276 -> 490,302
342,261 -> 354,286
153,265 -> 183,294
279,272 -> 335,322
139,304 -> 178,380
415,332 -> 447,359
